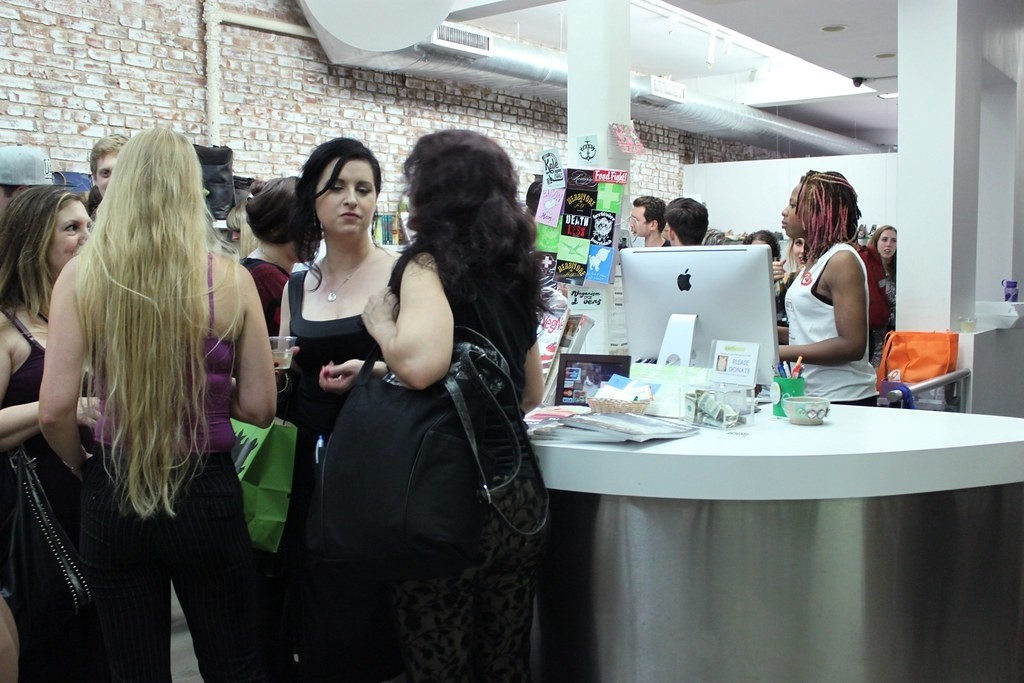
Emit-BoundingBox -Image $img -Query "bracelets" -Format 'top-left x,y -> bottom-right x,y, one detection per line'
62,445 -> 86,471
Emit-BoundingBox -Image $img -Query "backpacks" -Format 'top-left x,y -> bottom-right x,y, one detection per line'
303,326 -> 552,571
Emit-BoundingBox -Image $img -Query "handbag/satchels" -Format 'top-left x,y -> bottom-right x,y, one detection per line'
0,446 -> 91,629
228,414 -> 293,548
877,329 -> 959,402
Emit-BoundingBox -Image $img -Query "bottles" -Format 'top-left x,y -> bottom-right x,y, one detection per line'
1001,278 -> 1018,301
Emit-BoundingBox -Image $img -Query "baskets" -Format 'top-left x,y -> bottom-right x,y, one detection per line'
588,392 -> 647,415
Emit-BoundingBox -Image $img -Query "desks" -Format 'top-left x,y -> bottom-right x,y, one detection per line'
530,403 -> 1024,683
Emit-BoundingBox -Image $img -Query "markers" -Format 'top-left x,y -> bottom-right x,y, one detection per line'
771,365 -> 780,377
791,356 -> 804,379
778,362 -> 786,377
783,360 -> 791,378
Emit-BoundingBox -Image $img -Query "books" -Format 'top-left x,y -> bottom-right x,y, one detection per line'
523,405 -> 700,444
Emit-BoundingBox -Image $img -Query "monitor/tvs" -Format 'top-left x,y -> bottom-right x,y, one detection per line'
619,244 -> 779,394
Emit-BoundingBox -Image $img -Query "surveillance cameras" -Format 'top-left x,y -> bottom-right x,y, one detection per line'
853,78 -> 863,87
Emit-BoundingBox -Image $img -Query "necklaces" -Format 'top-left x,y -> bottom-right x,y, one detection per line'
325,273 -> 353,302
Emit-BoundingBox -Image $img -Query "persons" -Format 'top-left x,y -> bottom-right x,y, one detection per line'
744,230 -> 806,326
362,129 -> 552,683
274,138 -> 402,683
0,140 -> 78,208
523,180 -> 543,218
84,133 -> 129,217
38,127 -> 277,683
227,176 -> 322,337
0,186 -> 101,683
664,198 -> 709,246
630,196 -> 671,247
778,171 -> 880,407
852,225 -> 897,371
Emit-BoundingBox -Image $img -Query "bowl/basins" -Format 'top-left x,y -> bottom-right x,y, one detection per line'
782,396 -> 830,425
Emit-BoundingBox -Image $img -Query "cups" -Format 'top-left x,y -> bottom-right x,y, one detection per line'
269,336 -> 298,369
959,316 -> 976,332
772,375 -> 804,419
